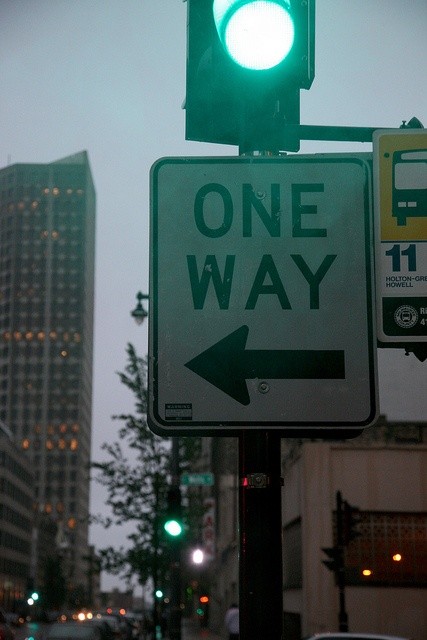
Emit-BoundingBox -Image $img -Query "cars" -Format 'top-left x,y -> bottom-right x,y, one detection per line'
0,608 -> 155,640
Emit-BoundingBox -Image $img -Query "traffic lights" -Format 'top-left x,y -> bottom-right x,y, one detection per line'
322,546 -> 341,579
343,502 -> 361,541
186,0 -> 315,153
30,592 -> 39,602
163,510 -> 182,551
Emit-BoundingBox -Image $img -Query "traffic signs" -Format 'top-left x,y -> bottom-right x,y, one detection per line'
146,154 -> 382,440
